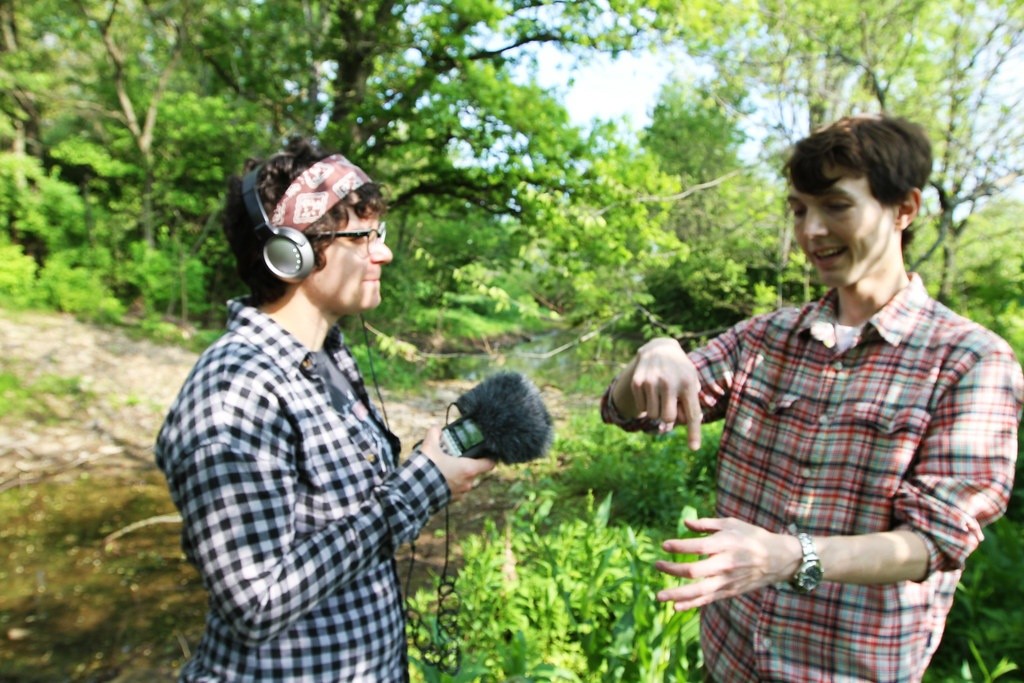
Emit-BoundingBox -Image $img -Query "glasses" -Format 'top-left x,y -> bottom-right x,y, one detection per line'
304,222 -> 387,255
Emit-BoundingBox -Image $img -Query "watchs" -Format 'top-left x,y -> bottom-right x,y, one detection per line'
789,532 -> 824,592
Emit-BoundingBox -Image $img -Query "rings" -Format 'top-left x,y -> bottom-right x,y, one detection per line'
658,419 -> 675,433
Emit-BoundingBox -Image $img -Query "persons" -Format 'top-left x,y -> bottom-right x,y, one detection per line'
156,134 -> 496,683
600,116 -> 1024,683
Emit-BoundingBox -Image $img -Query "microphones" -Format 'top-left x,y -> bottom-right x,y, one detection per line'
412,372 -> 555,467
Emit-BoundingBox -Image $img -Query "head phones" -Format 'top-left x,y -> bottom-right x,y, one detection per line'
241,152 -> 315,285
